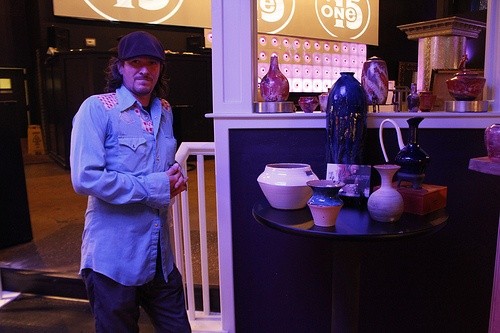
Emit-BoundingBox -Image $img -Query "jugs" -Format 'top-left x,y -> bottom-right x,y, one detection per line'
379,116 -> 431,189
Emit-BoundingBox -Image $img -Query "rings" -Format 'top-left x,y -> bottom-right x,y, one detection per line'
170,166 -> 177,174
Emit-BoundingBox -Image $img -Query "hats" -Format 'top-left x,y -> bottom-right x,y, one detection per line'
117,31 -> 166,62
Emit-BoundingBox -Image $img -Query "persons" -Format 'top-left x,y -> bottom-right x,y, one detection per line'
70,30 -> 192,333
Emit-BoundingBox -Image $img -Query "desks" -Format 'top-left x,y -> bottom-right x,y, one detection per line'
251,198 -> 450,333
43,48 -> 212,169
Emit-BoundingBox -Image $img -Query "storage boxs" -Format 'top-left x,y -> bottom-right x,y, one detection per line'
373,181 -> 448,216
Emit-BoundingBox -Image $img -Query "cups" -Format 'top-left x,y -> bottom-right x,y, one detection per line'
418,91 -> 434,111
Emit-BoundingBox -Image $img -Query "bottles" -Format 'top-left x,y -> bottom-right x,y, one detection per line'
260,56 -> 289,102
407,82 -> 420,112
326,72 -> 367,167
306,180 -> 345,227
368,165 -> 404,222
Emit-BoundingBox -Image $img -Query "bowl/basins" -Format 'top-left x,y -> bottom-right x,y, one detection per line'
298,97 -> 319,113
257,163 -> 320,208
